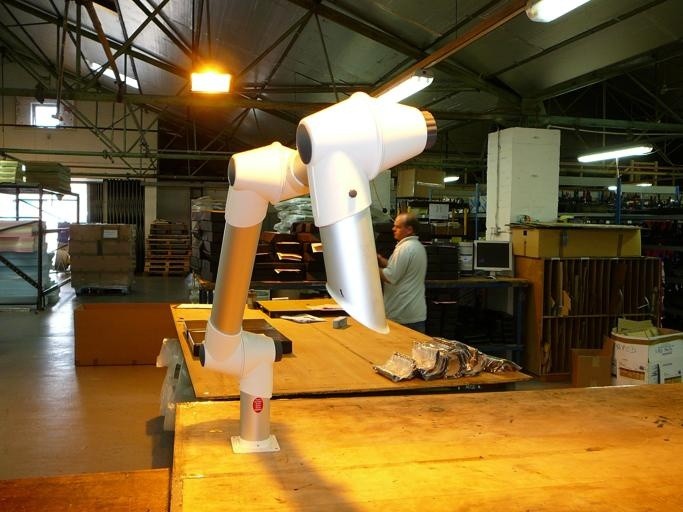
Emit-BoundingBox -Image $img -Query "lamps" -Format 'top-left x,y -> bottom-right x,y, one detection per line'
372,68 -> 434,104
576,141 -> 653,164
523,0 -> 590,23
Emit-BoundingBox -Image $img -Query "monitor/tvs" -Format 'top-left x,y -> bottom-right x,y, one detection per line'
472,239 -> 514,278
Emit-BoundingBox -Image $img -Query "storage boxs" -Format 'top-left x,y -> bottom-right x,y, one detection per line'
76,295 -> 180,366
570,349 -> 614,389
609,324 -> 682,384
68,223 -> 135,287
512,223 -> 641,258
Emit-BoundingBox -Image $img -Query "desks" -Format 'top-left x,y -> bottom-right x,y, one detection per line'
424,275 -> 530,368
0,468 -> 171,512
169,381 -> 683,512
169,298 -> 533,401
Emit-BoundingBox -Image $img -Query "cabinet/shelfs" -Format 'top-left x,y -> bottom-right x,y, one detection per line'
515,255 -> 664,382
143,235 -> 192,276
559,197 -> 683,321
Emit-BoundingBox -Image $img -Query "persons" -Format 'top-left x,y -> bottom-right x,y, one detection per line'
377,213 -> 428,336
